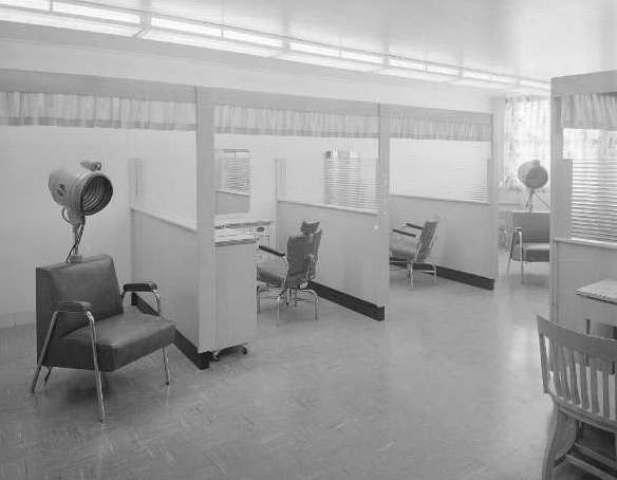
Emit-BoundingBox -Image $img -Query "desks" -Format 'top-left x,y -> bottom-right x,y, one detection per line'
576,278 -> 616,367
213,215 -> 273,360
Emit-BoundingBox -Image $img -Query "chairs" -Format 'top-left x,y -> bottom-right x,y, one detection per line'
257,218 -> 323,321
390,219 -> 439,288
535,312 -> 617,479
29,255 -> 175,421
505,212 -> 550,282
